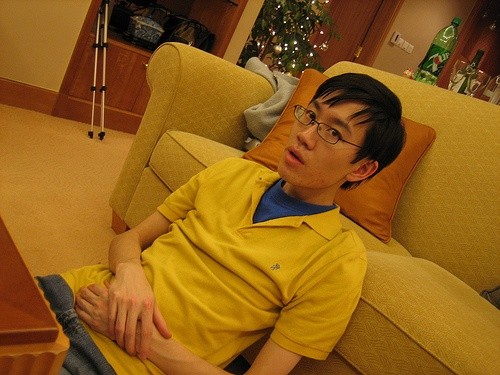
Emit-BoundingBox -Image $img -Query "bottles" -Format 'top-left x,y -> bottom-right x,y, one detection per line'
414,17 -> 461,87
481,73 -> 500,106
455,49 -> 485,94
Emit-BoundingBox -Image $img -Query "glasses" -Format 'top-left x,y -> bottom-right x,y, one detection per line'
294,104 -> 363,149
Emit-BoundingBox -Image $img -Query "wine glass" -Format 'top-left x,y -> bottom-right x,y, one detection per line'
465,68 -> 492,97
449,59 -> 470,91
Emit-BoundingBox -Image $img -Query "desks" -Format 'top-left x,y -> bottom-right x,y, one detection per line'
0,216 -> 72,375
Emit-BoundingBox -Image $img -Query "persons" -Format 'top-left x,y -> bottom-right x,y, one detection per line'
34,72 -> 407,374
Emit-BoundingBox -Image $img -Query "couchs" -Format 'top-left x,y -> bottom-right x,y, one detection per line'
108,40 -> 500,375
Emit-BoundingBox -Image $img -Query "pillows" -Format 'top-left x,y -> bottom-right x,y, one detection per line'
241,68 -> 437,244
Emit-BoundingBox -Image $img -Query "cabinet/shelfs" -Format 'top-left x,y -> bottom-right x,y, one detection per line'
66,33 -> 157,136
